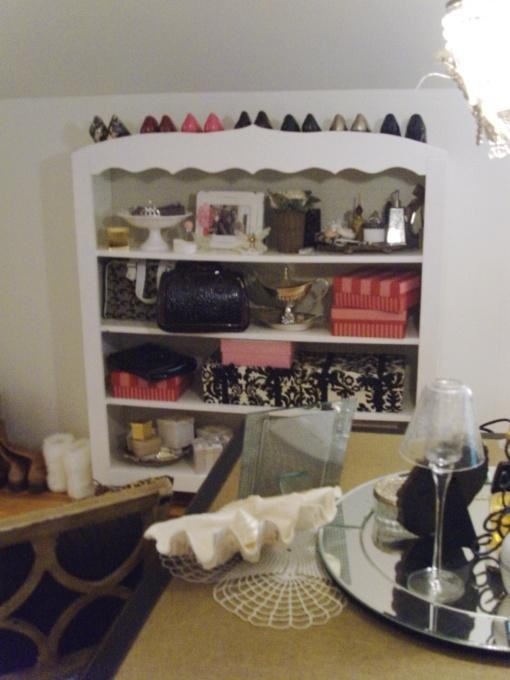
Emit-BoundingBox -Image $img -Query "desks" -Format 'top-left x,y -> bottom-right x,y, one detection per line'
89,411 -> 508,678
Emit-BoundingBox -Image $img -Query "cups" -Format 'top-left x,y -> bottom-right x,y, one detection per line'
41,432 -> 95,498
102,217 -> 130,251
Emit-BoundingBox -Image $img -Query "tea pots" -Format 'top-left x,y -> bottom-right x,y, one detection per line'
256,267 -> 330,324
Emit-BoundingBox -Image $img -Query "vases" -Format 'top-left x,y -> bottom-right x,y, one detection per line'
272,207 -> 323,254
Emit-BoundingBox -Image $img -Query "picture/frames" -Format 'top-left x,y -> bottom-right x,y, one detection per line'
195,192 -> 266,254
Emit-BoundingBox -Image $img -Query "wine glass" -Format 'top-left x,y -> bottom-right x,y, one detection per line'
117,209 -> 194,252
401,377 -> 479,604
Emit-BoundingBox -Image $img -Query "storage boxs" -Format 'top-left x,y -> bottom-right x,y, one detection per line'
329,265 -> 421,341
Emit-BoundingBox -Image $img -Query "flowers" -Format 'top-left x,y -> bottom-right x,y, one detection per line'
270,188 -> 321,212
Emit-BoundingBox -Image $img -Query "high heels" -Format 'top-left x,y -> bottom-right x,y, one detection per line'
87,115 -> 109,145
107,114 -> 131,139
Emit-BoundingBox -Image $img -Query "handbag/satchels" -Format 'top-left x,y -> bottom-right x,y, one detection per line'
154,264 -> 253,336
101,257 -> 176,322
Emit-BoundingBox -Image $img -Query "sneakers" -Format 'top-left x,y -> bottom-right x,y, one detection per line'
140,111 -> 427,143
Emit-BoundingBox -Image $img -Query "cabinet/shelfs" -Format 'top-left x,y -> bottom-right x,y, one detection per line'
70,124 -> 444,493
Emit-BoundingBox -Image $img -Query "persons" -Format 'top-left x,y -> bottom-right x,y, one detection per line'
208,205 -> 247,234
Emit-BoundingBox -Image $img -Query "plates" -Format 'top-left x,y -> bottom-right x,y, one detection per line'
116,440 -> 194,467
256,310 -> 324,330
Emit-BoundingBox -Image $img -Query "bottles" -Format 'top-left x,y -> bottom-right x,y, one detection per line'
354,200 -> 409,246
